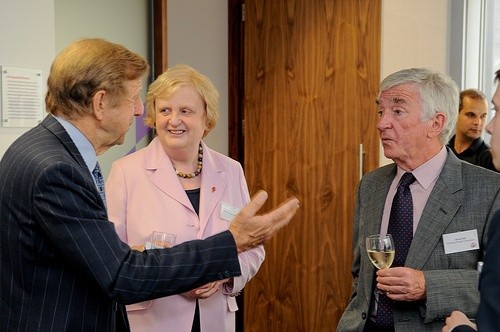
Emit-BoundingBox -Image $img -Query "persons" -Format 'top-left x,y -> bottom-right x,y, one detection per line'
334,67 -> 499,331
445,90 -> 499,175
104,65 -> 266,332
441,69 -> 499,332
0,38 -> 300,332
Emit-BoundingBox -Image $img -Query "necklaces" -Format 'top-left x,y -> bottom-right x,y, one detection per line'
173,143 -> 203,179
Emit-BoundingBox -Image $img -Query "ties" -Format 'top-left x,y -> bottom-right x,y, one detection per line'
374,171 -> 414,332
92,161 -> 107,210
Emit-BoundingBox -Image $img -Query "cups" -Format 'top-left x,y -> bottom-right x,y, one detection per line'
151,231 -> 176,250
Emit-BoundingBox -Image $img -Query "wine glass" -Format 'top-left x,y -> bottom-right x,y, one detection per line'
366,234 -> 396,294
